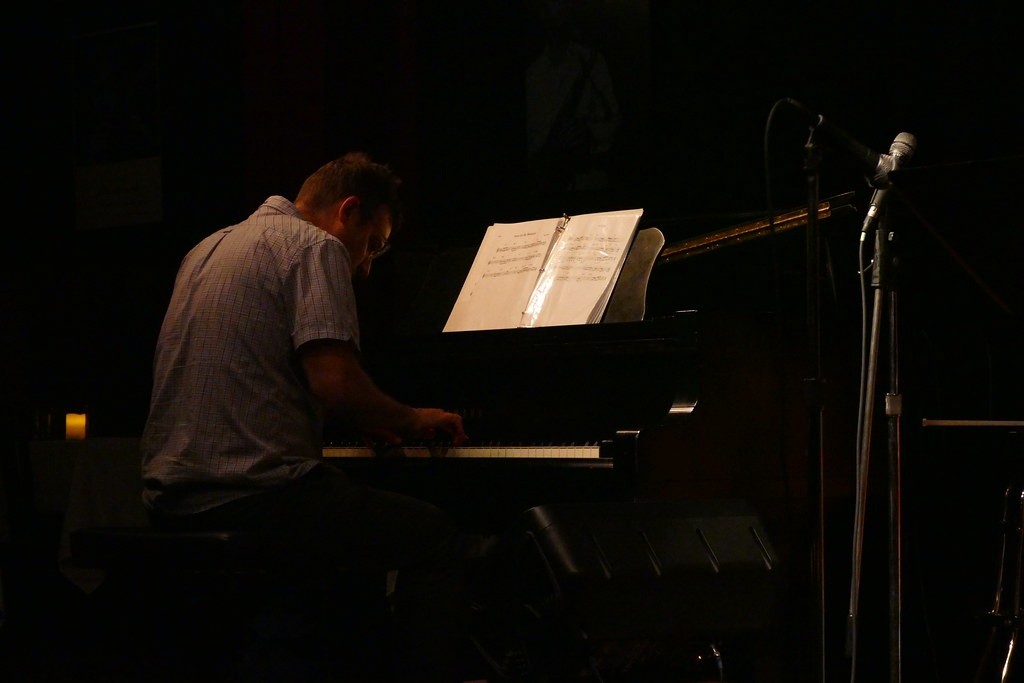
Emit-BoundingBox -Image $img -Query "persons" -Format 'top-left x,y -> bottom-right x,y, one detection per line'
140,150 -> 470,683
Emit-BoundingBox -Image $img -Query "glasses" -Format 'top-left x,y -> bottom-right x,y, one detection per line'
362,222 -> 389,258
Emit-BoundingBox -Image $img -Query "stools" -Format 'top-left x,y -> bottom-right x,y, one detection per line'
68,530 -> 298,683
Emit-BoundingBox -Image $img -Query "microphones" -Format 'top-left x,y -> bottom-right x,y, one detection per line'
861,132 -> 914,235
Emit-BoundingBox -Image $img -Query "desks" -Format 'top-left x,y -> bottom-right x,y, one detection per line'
29,438 -> 145,526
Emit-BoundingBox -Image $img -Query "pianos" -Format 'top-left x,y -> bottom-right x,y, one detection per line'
315,206 -> 670,599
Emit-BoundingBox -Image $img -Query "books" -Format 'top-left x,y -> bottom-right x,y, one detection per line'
441,207 -> 644,332
